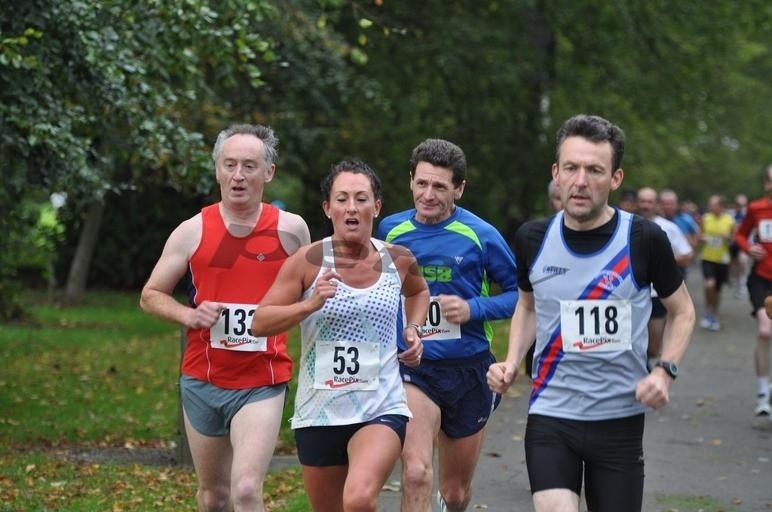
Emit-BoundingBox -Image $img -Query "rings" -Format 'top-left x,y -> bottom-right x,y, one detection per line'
446,311 -> 448,318
330,281 -> 333,286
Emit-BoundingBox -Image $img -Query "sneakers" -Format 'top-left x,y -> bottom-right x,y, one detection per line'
699,314 -> 722,331
754,394 -> 771,416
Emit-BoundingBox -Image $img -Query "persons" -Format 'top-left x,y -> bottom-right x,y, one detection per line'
251,160 -> 430,512
735,160 -> 772,416
485,113 -> 696,512
138,122 -> 311,512
526,179 -> 561,378
619,185 -> 754,372
378,139 -> 519,512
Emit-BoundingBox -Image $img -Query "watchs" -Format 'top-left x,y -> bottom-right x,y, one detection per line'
656,361 -> 679,379
405,323 -> 425,337
